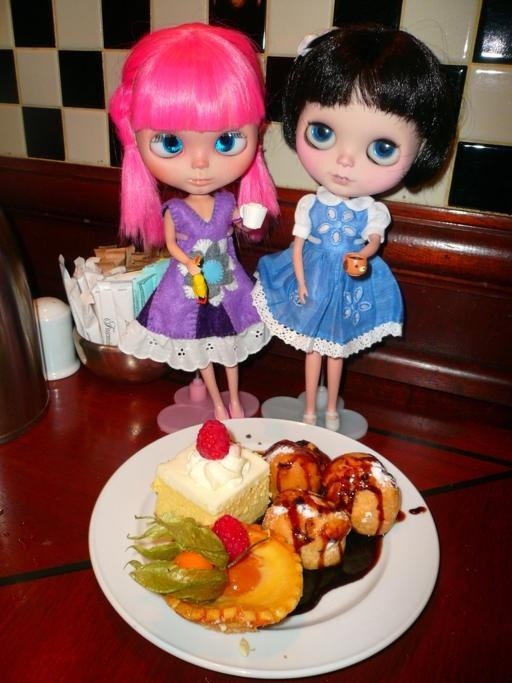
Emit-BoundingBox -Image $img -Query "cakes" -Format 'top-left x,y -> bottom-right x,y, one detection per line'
153,418 -> 270,531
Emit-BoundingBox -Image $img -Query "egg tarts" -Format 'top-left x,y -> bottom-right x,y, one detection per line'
162,523 -> 304,629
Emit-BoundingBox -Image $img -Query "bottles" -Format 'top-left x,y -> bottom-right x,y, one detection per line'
34,298 -> 81,381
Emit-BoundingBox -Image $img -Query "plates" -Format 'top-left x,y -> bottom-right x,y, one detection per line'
89,416 -> 443,678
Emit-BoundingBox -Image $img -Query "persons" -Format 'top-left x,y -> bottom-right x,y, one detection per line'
253,26 -> 450,430
108,21 -> 281,421
